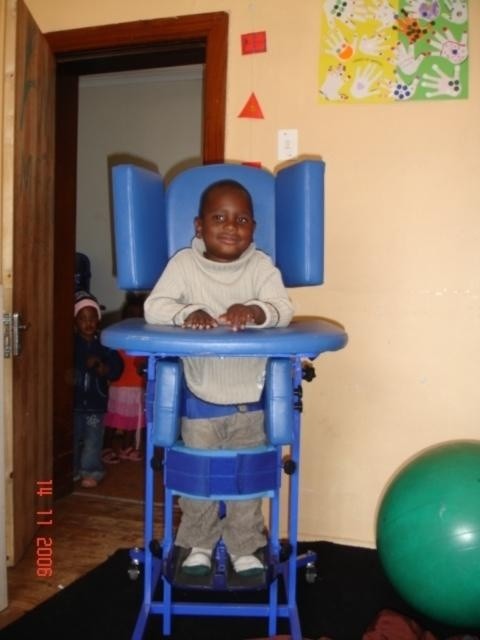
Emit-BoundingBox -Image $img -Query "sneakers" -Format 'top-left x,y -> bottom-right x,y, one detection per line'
81,477 -> 98,489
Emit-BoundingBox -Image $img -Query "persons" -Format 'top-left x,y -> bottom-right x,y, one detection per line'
138,178 -> 296,581
67,289 -> 128,491
100,288 -> 149,466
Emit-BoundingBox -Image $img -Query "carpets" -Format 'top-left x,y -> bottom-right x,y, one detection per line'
1,541 -> 386,634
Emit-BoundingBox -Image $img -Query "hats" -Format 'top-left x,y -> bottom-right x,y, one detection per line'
73,290 -> 101,320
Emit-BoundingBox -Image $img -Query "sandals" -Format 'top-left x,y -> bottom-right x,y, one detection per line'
101,445 -> 143,465
